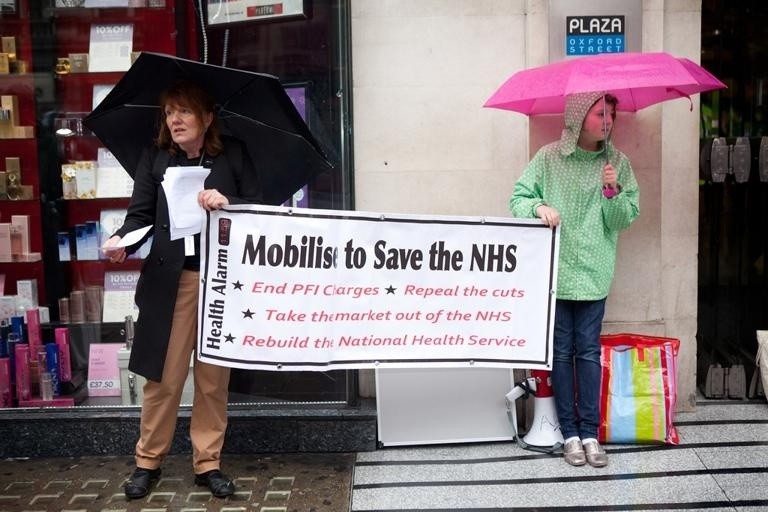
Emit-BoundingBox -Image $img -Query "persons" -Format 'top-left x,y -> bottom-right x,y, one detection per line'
511,93 -> 639,470
98,82 -> 238,501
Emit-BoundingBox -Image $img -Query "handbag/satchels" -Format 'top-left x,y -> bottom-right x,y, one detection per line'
570,332 -> 680,446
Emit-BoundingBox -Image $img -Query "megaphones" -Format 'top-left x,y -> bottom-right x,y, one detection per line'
505,369 -> 566,447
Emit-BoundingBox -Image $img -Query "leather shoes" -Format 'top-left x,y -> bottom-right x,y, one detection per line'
124,465 -> 162,500
193,468 -> 236,498
563,436 -> 586,466
585,436 -> 609,469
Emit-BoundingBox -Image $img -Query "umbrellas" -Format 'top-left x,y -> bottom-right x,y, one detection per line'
483,51 -> 730,197
80,51 -> 332,205
